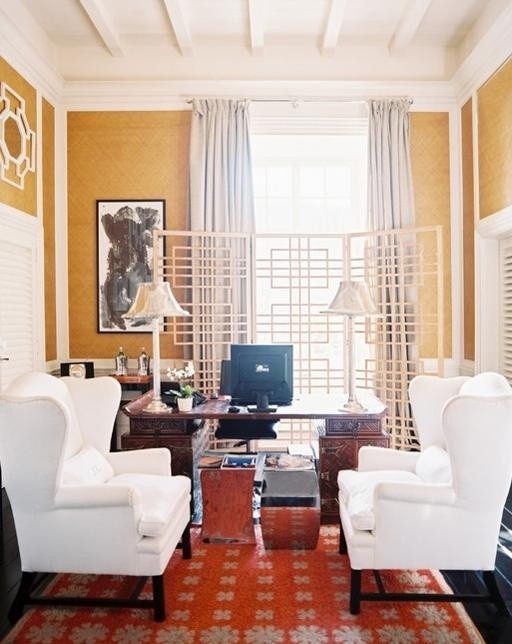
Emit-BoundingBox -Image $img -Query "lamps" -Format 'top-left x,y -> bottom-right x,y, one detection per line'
321,282 -> 383,413
121,281 -> 191,414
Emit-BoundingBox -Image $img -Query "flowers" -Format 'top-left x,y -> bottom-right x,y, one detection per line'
167,360 -> 195,403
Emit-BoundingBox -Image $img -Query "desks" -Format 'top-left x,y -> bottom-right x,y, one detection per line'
51,366 -> 182,393
121,388 -> 389,526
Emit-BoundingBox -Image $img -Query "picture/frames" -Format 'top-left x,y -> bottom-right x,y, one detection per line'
95,200 -> 167,335
60,362 -> 94,379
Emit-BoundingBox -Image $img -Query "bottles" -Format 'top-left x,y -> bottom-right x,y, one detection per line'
115,345 -> 129,376
137,346 -> 151,376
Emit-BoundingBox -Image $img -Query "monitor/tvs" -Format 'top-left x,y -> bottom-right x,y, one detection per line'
230,344 -> 294,411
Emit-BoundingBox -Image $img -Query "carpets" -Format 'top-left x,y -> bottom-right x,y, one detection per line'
2,523 -> 486,644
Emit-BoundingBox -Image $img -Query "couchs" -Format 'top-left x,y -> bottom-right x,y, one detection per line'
336,372 -> 512,615
0,370 -> 192,623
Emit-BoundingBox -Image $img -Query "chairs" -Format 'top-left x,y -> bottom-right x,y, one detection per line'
216,360 -> 280,455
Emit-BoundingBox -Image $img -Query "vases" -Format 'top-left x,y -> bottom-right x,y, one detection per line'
177,397 -> 193,411
